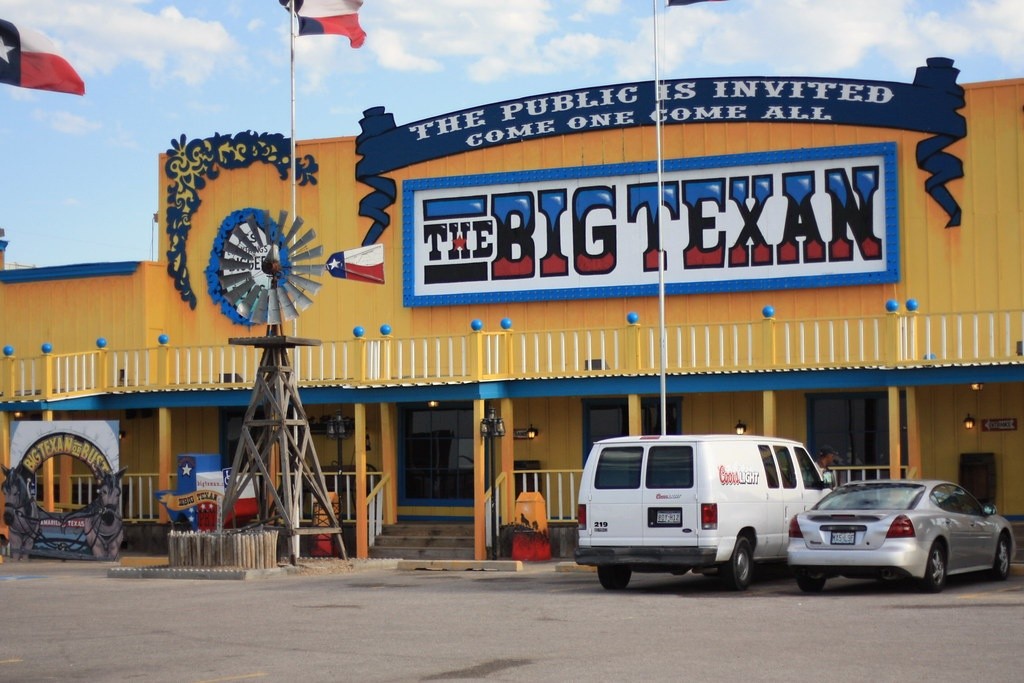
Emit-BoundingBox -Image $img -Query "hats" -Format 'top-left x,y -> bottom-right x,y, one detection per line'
820,444 -> 839,457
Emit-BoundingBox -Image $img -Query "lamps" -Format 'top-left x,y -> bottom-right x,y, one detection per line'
735,420 -> 746,434
970,383 -> 984,391
426,401 -> 440,409
527,424 -> 538,439
964,413 -> 975,430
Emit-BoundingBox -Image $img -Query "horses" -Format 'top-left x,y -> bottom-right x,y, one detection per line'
84,464 -> 129,562
0,463 -> 40,561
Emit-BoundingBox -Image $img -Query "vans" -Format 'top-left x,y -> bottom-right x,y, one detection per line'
576,434 -> 840,592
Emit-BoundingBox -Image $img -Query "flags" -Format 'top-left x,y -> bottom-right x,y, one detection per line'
280,1 -> 366,48
665,0 -> 730,7
0,19 -> 84,95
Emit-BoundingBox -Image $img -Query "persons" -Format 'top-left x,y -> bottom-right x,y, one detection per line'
818,446 -> 845,484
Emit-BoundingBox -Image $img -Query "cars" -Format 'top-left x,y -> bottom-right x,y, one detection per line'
785,478 -> 1018,594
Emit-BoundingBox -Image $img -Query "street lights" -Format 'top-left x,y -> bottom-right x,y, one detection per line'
327,409 -> 349,559
482,405 -> 507,559
